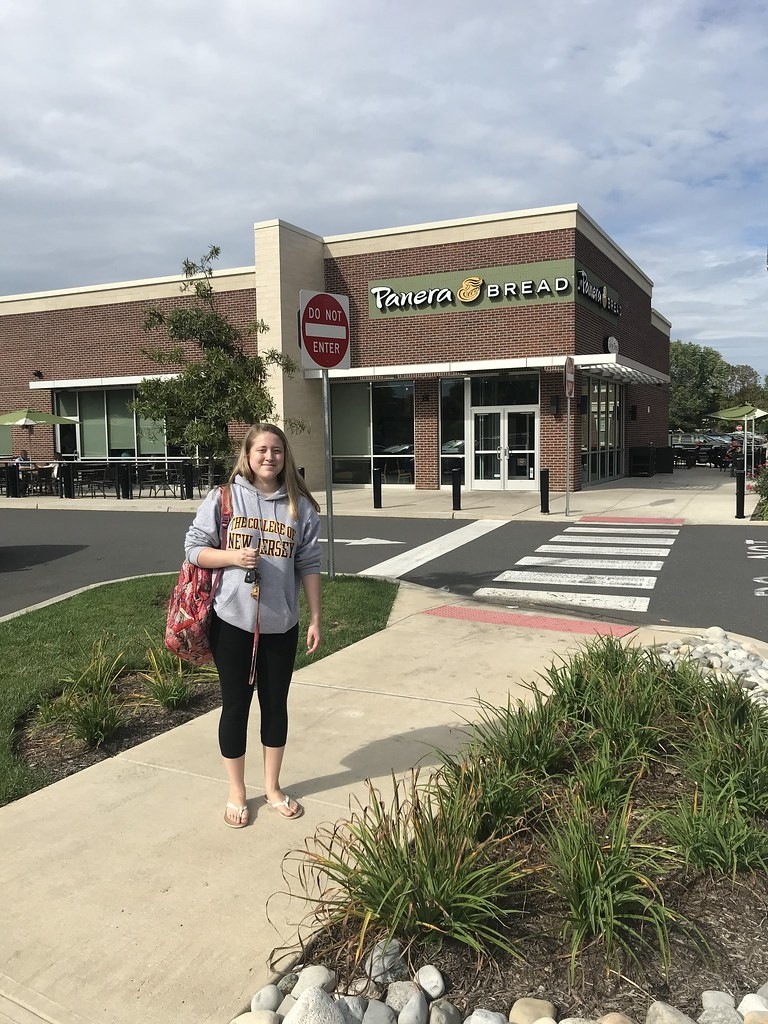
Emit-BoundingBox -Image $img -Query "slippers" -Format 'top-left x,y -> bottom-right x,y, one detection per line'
223,802 -> 249,828
263,794 -> 303,819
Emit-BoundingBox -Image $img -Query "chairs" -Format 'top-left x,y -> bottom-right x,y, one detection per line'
174,465 -> 202,500
35,465 -> 67,495
73,465 -> 91,497
135,466 -> 156,499
93,467 -> 119,499
672,443 -> 743,472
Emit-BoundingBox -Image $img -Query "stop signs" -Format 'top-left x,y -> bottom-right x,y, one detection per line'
299,284 -> 349,371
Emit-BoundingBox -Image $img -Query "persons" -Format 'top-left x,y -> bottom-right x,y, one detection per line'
182,424 -> 322,828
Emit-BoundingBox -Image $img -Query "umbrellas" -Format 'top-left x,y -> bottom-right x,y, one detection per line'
0,408 -> 83,482
704,402 -> 768,456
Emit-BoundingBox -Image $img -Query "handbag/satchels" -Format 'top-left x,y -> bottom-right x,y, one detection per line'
165,559 -> 213,665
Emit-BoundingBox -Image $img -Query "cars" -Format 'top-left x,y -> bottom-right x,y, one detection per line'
667,430 -> 768,467
364,431 -> 534,480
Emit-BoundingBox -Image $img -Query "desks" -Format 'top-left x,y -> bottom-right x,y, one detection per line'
19,466 -> 53,495
78,469 -> 106,499
146,468 -> 179,497
